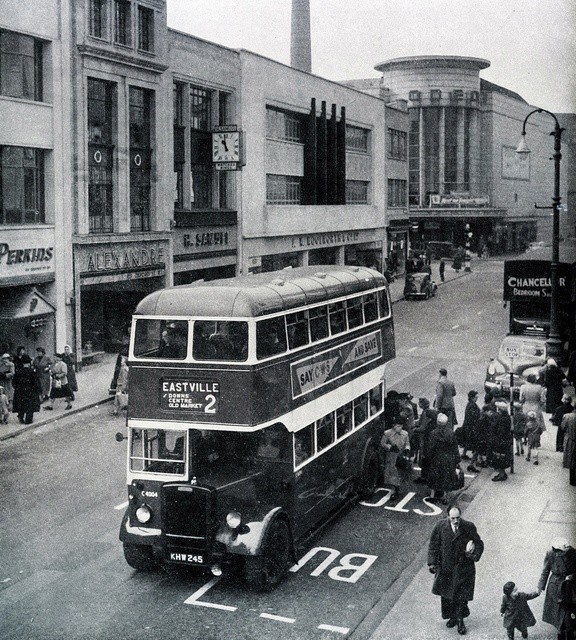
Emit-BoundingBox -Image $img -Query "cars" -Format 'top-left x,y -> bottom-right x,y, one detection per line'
483,334 -> 558,408
402,271 -> 437,301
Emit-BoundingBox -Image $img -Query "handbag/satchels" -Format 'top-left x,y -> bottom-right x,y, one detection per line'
118,394 -> 128,408
455,465 -> 465,488
397,454 -> 411,471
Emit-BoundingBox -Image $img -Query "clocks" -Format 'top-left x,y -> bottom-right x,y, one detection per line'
211,131 -> 240,162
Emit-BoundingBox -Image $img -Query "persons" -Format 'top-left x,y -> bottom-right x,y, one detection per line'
461,390 -> 481,460
487,400 -> 514,482
380,419 -> 411,500
539,537 -> 576,640
386,389 -> 433,419
561,398 -> 576,487
61,346 -> 78,401
546,359 -> 567,421
427,506 -> 484,635
522,410 -> 544,465
13,344 -> 31,366
42,353 -> 74,411
511,402 -> 526,456
0,354 -> 14,406
467,405 -> 495,474
405,244 -> 462,282
31,347 -> 50,401
0,386 -> 10,425
549,394 -> 575,452
519,373 -> 546,435
434,368 -> 458,425
498,581 -> 540,639
424,413 -> 465,506
11,354 -> 42,424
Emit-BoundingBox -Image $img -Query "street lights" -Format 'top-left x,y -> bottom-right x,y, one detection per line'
514,108 -> 567,388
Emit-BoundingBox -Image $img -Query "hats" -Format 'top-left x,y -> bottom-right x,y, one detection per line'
495,400 -> 508,410
552,539 -> 570,553
437,413 -> 448,424
560,393 -> 571,404
1,354 -> 10,358
468,390 -> 478,400
503,582 -> 515,594
36,347 -> 45,354
390,415 -> 405,424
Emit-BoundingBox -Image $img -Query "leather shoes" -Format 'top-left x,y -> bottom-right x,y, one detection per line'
456,618 -> 466,635
446,618 -> 458,628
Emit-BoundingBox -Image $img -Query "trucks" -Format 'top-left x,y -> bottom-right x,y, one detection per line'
502,259 -> 571,337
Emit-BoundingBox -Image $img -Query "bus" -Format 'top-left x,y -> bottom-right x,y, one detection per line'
113,262 -> 397,593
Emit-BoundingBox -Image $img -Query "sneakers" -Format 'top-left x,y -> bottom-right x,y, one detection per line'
0,419 -> 4,425
525,457 -> 530,461
476,460 -> 486,467
64,397 -> 74,402
43,406 -> 54,411
17,415 -> 24,424
426,497 -> 437,503
521,450 -> 523,454
467,465 -> 479,473
24,419 -> 33,424
3,420 -> 8,425
65,405 -> 72,409
390,493 -> 398,500
460,455 -> 472,460
491,474 -> 507,481
533,461 -> 538,464
515,452 -> 520,455
